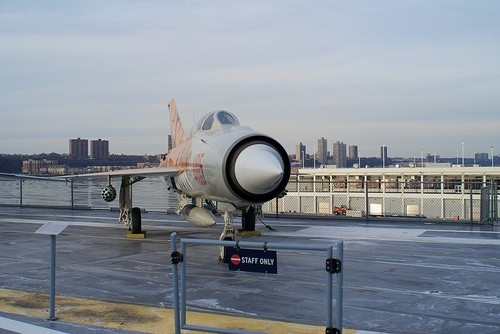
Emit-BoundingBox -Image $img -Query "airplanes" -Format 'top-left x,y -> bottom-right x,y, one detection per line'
57,97 -> 387,263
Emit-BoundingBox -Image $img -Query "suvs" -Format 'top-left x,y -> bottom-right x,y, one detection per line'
333,204 -> 352,216
